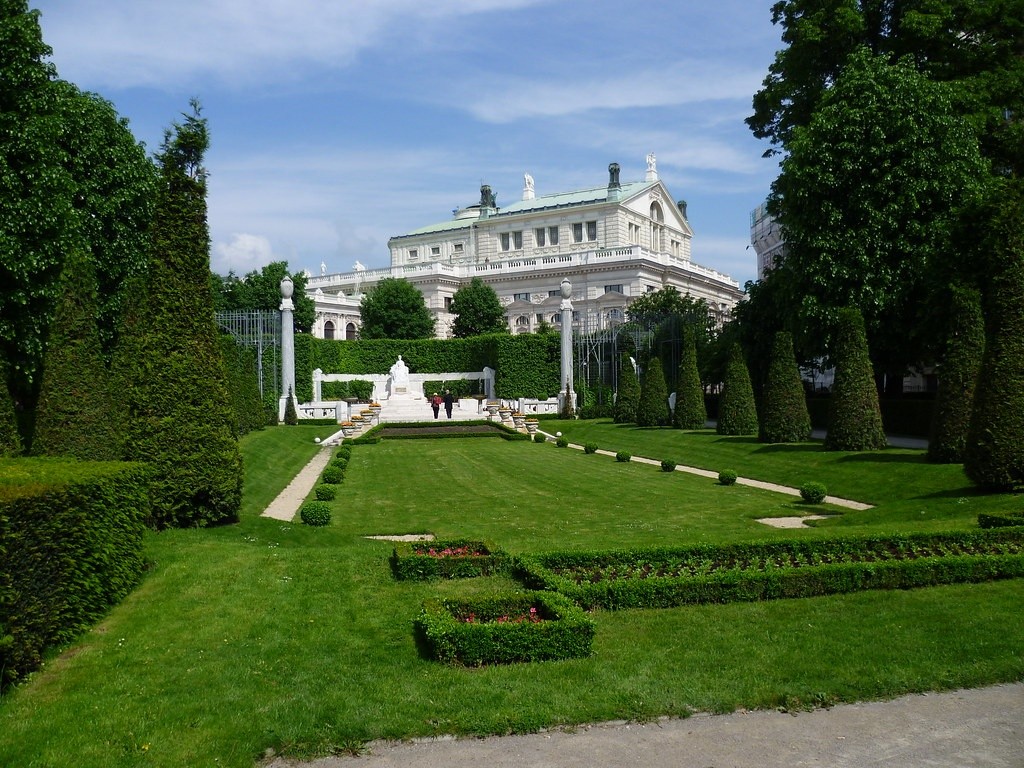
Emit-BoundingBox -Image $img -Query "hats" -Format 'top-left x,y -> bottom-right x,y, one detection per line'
433,393 -> 438,396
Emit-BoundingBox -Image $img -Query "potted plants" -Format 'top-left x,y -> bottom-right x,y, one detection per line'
486,402 -> 499,416
360,410 -> 376,425
368,401 -> 382,420
341,422 -> 356,438
350,415 -> 365,432
523,418 -> 539,434
512,412 -> 526,428
498,406 -> 513,422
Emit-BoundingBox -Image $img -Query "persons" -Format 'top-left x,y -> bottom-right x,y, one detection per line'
431,393 -> 442,419
443,390 -> 453,418
396,355 -> 405,369
